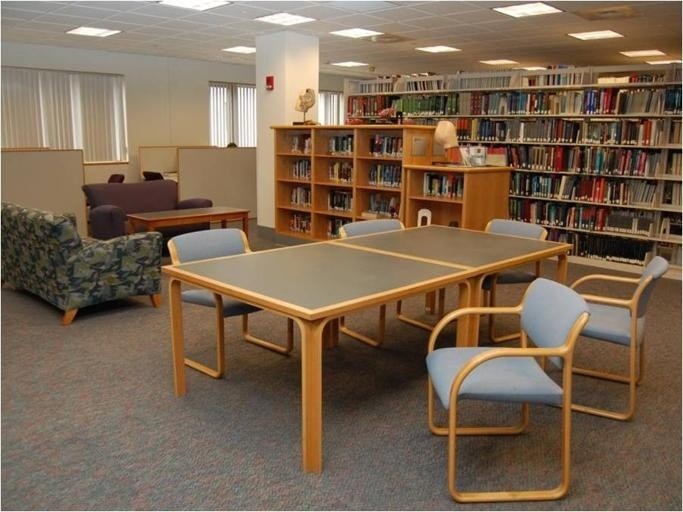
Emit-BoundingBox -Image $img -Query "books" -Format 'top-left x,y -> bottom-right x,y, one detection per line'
328,134 -> 354,236
291,134 -> 311,232
509,198 -> 682,265
372,117 -> 682,145
423,172 -> 463,197
510,173 -> 681,206
460,142 -> 682,177
349,63 -> 681,114
368,136 -> 402,216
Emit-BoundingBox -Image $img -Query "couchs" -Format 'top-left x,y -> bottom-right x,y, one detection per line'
2,202 -> 163,326
80,179 -> 214,257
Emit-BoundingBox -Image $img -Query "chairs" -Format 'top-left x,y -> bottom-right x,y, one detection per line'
108,174 -> 124,184
143,172 -> 164,180
